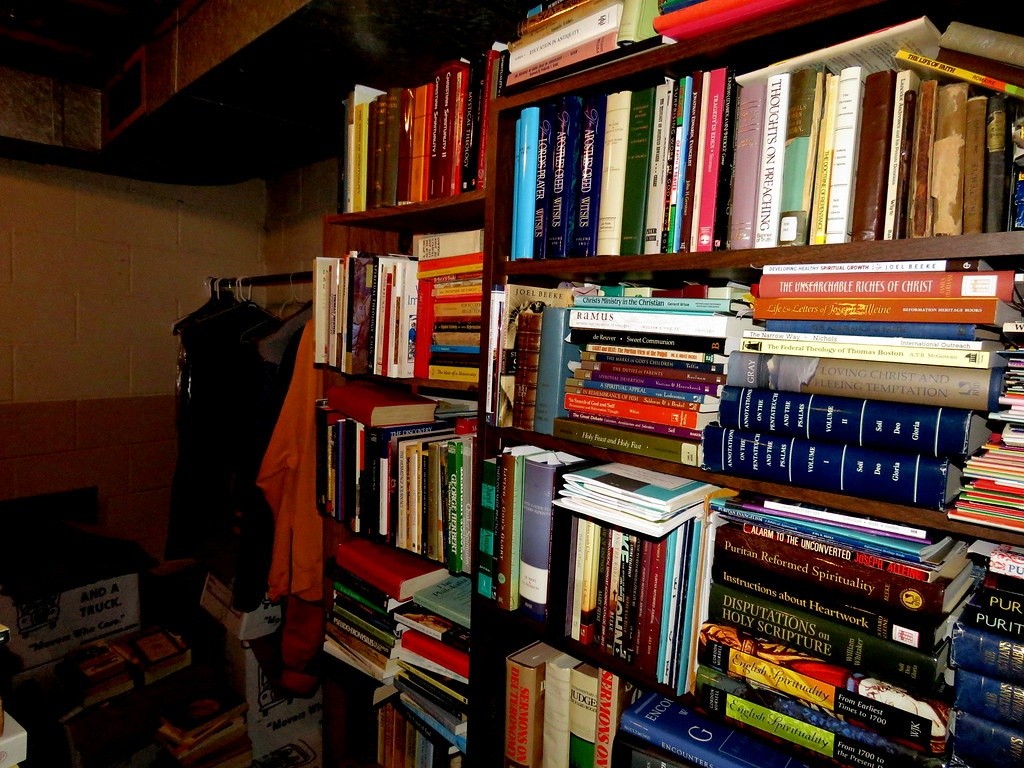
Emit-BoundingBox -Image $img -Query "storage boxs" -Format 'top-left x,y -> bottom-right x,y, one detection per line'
199,572 -> 288,642
0,568 -> 140,673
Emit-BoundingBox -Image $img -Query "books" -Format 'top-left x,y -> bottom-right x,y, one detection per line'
316,383 -> 478,576
1024,607 -> 1024,609
79,644 -> 135,708
510,17 -> 1024,261
484,262 -> 1024,534
343,43 -> 506,214
313,229 -> 484,383
123,629 -> 192,686
508,0 -> 879,93
322,538 -> 472,768
476,445 -> 1024,768
154,687 -> 254,768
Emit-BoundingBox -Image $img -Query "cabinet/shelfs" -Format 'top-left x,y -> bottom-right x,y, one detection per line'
322,0 -> 1024,768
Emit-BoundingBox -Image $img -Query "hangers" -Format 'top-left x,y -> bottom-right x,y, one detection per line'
232,276 -> 273,323
274,271 -> 308,320
207,276 -> 229,300
